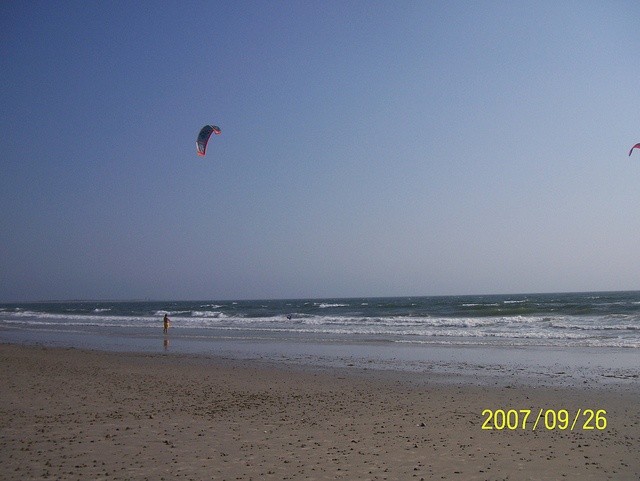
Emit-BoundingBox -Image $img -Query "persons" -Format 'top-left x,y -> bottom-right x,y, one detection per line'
163,313 -> 170,334
